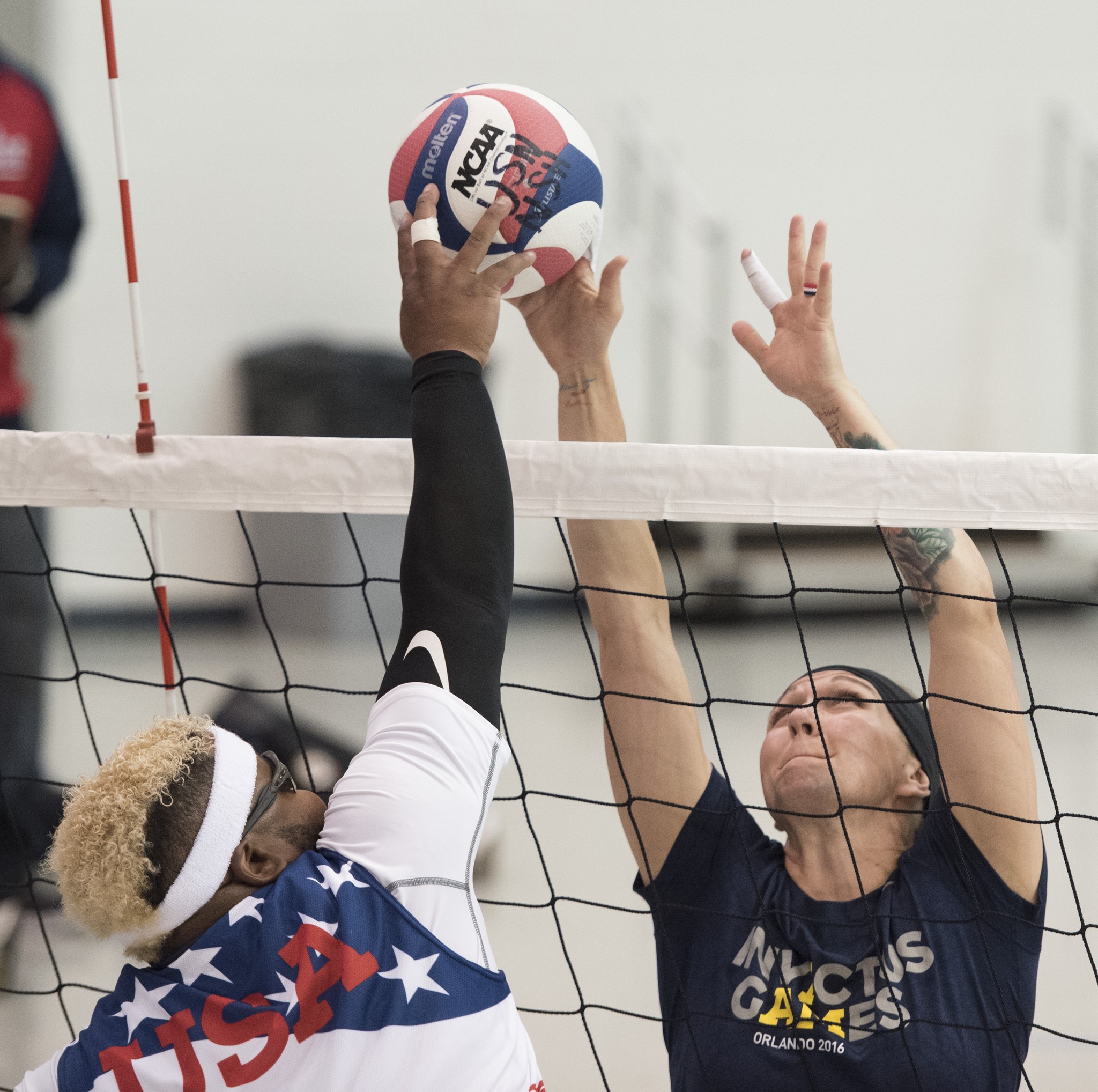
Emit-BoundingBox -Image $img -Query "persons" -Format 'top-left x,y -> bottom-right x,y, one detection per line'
11,183 -> 538,1092
506,215 -> 1048,1092
0,53 -> 86,911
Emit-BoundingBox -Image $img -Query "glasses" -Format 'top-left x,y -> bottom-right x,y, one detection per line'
240,747 -> 297,851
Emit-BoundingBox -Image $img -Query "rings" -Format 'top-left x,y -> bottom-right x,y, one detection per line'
803,283 -> 817,295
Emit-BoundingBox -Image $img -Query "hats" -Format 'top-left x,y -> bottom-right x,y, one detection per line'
802,666 -> 945,824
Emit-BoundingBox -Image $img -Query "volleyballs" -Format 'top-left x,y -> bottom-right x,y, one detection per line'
388,82 -> 604,300
